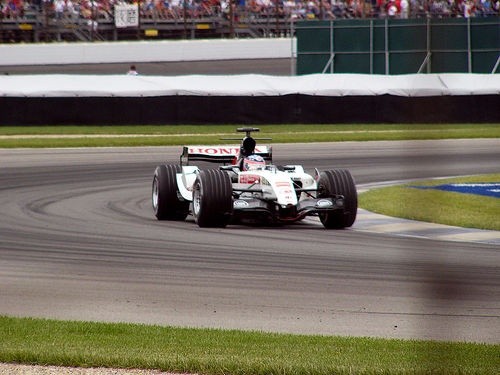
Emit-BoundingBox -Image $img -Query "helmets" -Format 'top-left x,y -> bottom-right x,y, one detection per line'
242,154 -> 264,172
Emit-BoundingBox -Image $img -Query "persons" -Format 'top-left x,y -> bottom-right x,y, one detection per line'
242,155 -> 266,171
125,65 -> 139,75
1,0 -> 500,31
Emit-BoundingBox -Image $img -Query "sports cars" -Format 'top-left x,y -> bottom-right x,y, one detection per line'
148,125 -> 359,229
